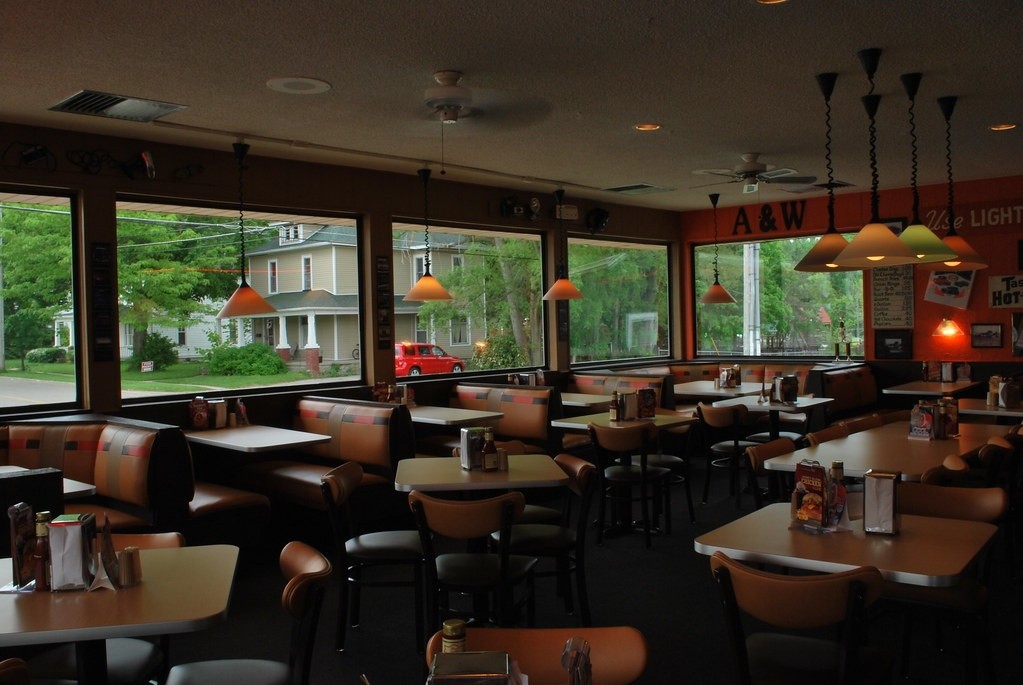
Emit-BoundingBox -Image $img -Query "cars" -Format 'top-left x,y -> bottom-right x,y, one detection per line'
394,344 -> 464,376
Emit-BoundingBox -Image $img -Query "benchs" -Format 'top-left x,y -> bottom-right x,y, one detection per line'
0,359 -> 904,581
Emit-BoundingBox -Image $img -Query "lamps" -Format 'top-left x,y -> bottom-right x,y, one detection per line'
219,142 -> 278,318
931,320 -> 964,336
795,47 -> 989,272
541,190 -> 585,300
403,169 -> 454,301
501,195 -> 541,219
700,193 -> 737,304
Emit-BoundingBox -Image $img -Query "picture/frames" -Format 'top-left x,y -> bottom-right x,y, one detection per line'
970,323 -> 1003,348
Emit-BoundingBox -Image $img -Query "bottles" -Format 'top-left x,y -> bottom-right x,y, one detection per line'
386,385 -> 396,404
714,378 -> 720,389
235,397 -> 245,424
31,510 -> 51,591
608,390 -> 625,422
922,360 -> 928,381
480,426 -> 498,472
727,367 -> 736,388
497,447 -> 509,471
912,398 -> 948,441
119,545 -> 143,588
506,374 -> 516,385
830,460 -> 850,526
986,376 -> 1014,406
229,413 -> 237,427
441,619 -> 467,652
769,376 -> 778,403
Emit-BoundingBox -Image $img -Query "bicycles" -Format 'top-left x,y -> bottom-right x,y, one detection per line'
352,343 -> 360,360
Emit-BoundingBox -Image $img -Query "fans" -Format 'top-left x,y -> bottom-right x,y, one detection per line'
686,152 -> 817,194
354,67 -> 559,176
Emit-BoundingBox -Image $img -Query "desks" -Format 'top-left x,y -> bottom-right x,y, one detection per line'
713,395 -> 835,499
561,392 -> 620,407
394,454 -> 569,628
551,412 -> 700,539
692,503 -> 999,587
673,380 -> 772,397
409,406 -> 504,426
181,421 -> 332,454
957,398 -> 1023,417
762,419 -> 1012,482
0,465 -> 96,500
0,544 -> 239,685
882,380 -> 981,396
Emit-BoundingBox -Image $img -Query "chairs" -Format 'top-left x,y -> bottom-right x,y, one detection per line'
0,404 -> 1023,685
